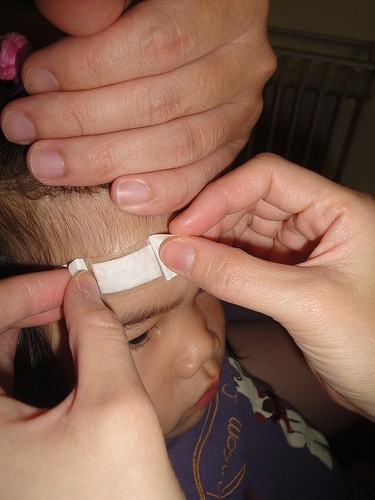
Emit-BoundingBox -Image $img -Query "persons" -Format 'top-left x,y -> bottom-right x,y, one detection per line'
0,0 -> 334,500
0,151 -> 375,500
0,1 -> 277,215
